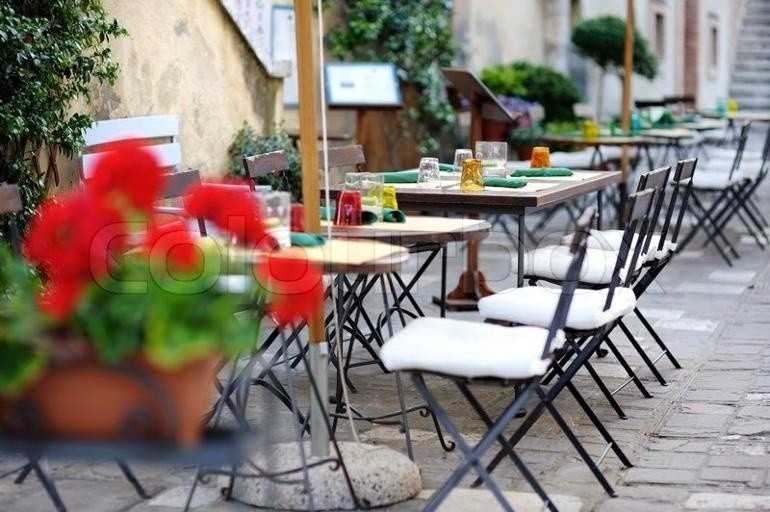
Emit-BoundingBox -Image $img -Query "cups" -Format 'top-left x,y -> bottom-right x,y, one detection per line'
459,159 -> 484,191
630,111 -> 640,135
261,193 -> 291,248
417,158 -> 441,192
715,97 -> 738,116
292,205 -> 303,231
452,150 -> 473,174
531,147 -> 549,167
583,119 -> 598,137
475,140 -> 505,180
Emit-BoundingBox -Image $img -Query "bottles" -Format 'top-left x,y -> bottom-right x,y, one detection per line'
332,173 -> 398,227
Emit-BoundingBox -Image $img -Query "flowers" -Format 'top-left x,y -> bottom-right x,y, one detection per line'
1,137 -> 330,395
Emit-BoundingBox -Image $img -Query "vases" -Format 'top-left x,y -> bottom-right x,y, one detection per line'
24,348 -> 229,450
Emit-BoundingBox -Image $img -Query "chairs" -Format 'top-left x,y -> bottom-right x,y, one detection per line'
158,93 -> 770,512
76,114 -> 275,293
